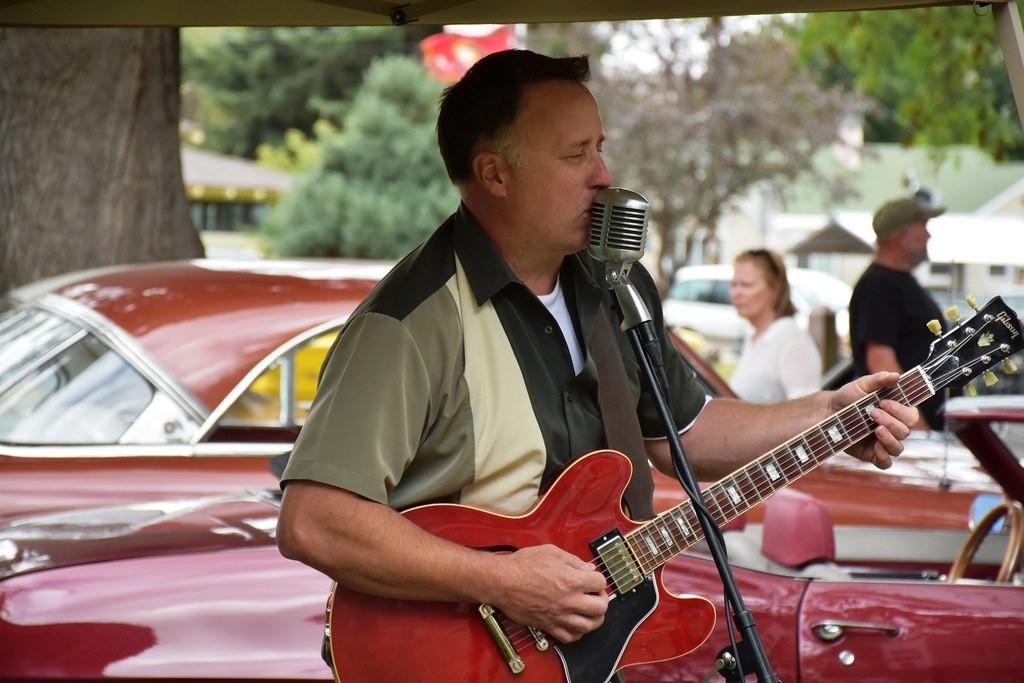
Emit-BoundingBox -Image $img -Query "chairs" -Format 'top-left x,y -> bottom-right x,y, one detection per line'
762,486 -> 851,582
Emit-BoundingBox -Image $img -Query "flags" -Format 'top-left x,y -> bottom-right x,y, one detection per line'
420,26 -> 511,82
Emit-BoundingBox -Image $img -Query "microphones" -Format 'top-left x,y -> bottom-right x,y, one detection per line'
587,185 -> 669,391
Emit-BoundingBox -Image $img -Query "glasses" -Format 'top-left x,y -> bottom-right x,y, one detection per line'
749,248 -> 780,276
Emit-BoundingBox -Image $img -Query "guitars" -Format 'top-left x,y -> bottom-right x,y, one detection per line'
321,290 -> 1024,683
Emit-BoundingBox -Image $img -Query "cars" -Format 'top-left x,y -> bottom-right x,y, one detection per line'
1,256 -> 1024,683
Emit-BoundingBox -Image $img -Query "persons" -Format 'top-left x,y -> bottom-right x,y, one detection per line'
849,199 -> 949,432
276,49 -> 919,683
731,249 -> 823,403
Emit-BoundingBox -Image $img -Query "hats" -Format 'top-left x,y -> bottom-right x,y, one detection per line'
872,197 -> 947,236
